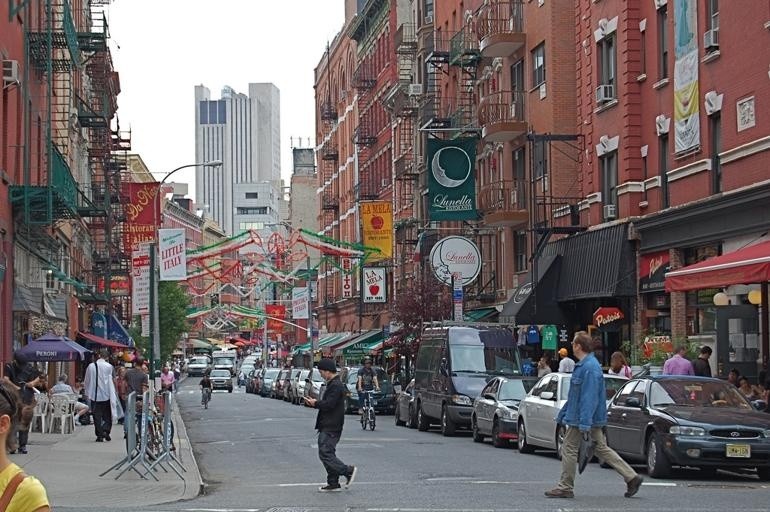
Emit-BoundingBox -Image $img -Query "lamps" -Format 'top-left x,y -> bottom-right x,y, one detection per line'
713,286 -> 729,306
747,288 -> 763,337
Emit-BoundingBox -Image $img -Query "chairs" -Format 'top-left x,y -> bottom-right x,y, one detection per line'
29,389 -> 81,436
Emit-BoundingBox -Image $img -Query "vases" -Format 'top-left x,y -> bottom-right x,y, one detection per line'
649,366 -> 664,377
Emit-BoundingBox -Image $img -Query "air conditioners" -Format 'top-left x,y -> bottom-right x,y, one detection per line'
2,59 -> 21,85
408,83 -> 423,96
602,204 -> 615,219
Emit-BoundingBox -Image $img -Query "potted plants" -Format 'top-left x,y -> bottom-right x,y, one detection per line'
620,326 -> 648,378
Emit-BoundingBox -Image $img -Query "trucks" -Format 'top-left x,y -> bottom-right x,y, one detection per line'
412,326 -> 528,438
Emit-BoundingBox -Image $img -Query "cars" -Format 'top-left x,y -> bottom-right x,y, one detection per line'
468,376 -> 544,449
303,367 -> 343,404
605,374 -> 770,481
338,365 -> 395,415
208,346 -> 315,408
515,371 -> 633,460
392,378 -> 417,425
358,389 -> 382,429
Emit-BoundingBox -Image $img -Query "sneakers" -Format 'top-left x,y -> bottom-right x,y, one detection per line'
345,465 -> 357,488
318,484 -> 341,491
624,476 -> 642,497
545,489 -> 574,498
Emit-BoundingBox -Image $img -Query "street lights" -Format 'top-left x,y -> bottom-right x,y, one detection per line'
263,219 -> 316,366
149,160 -> 224,417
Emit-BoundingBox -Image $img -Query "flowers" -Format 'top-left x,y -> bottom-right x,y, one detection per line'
643,340 -> 674,367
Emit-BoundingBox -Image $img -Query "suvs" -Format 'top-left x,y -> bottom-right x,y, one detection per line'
187,357 -> 210,376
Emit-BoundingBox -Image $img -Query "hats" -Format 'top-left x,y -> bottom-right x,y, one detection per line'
317,360 -> 336,370
558,348 -> 567,353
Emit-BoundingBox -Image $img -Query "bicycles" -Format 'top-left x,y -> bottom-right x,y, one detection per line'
201,388 -> 211,409
120,390 -> 178,458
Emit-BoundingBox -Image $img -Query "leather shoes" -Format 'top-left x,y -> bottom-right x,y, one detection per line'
102,432 -> 111,441
95,437 -> 103,442
18,447 -> 27,454
